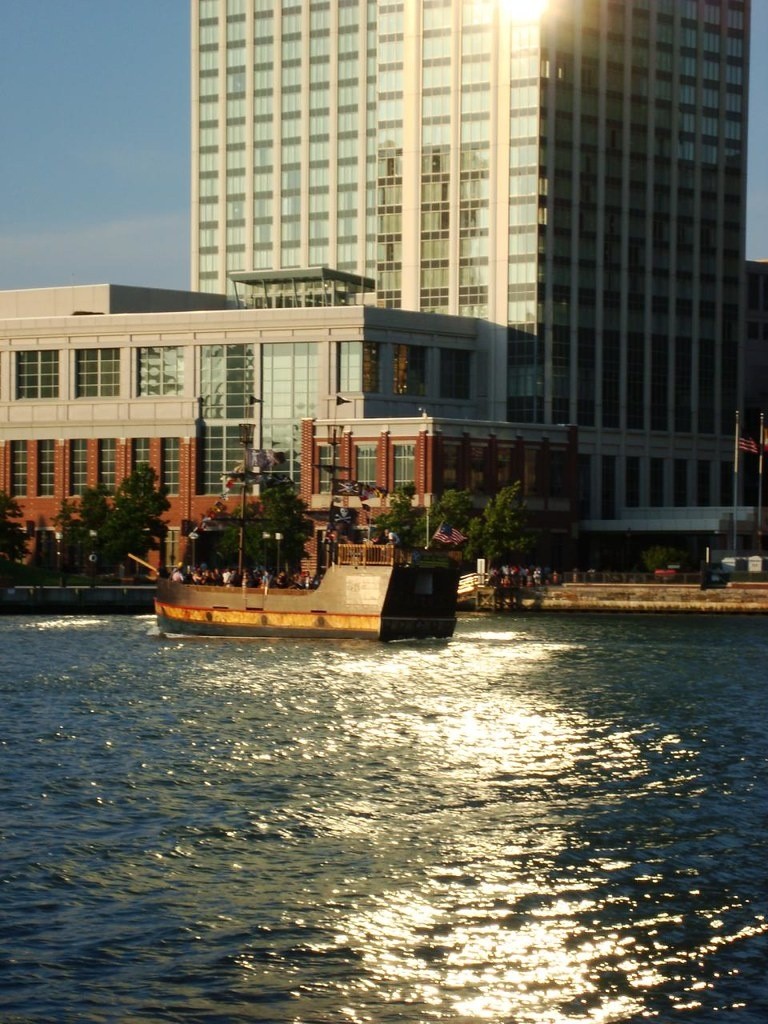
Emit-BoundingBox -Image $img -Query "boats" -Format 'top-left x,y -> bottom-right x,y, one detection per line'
150,389 -> 461,642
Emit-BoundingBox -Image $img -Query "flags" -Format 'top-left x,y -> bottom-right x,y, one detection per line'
187,467 -> 241,541
434,522 -> 468,549
738,435 -> 759,453
365,484 -> 387,499
246,449 -> 286,467
329,506 -> 358,526
763,429 -> 768,450
332,479 -> 363,496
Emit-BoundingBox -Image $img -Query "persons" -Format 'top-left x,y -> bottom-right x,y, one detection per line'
488,563 -> 560,588
588,568 -> 596,583
383,529 -> 401,547
572,567 -> 578,583
170,565 -> 323,590
